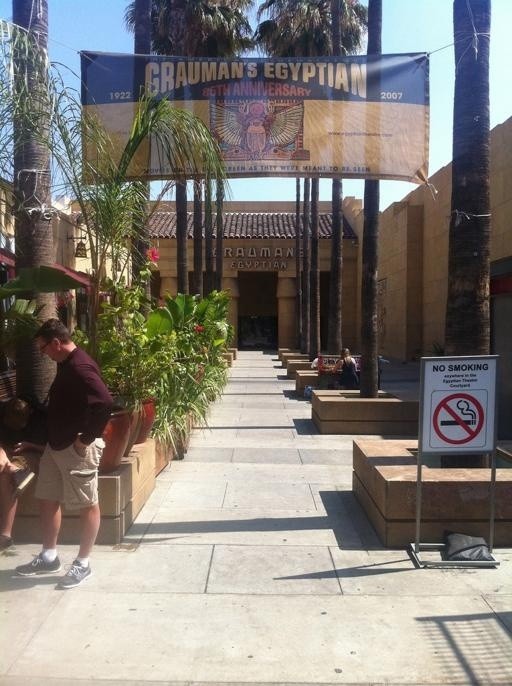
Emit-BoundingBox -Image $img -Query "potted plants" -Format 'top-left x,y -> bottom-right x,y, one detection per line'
71,248 -> 180,475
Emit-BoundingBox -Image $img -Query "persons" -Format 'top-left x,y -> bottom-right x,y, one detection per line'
238,100 -> 276,158
1,398 -> 49,550
12,317 -> 114,591
311,352 -> 322,370
330,347 -> 359,388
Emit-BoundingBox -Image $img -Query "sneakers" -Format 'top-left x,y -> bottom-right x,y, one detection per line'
0,533 -> 17,552
58,559 -> 90,588
16,552 -> 60,575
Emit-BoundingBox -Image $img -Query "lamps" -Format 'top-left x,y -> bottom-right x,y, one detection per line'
67,237 -> 87,258
